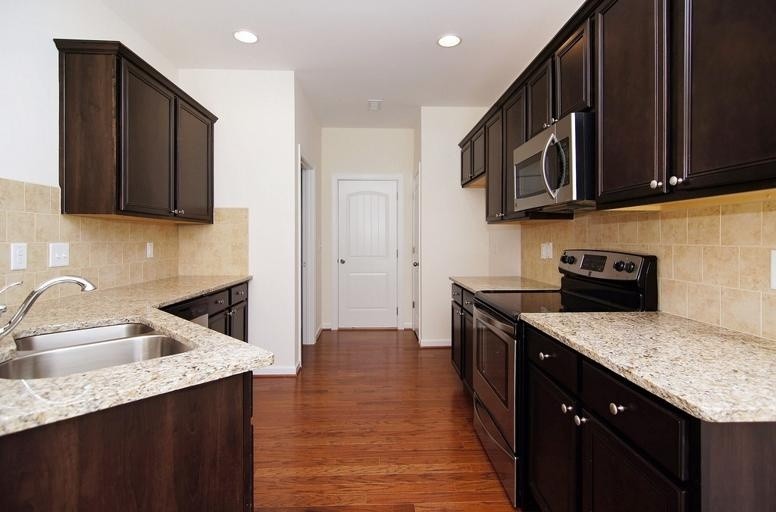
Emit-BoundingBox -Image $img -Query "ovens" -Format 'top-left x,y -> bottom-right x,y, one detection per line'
470,306 -> 520,511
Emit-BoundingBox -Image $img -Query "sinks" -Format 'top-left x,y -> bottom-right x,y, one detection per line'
0,330 -> 202,380
11,315 -> 157,352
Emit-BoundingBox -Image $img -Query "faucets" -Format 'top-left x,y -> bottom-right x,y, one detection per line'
0,274 -> 98,342
21,379 -> 92,405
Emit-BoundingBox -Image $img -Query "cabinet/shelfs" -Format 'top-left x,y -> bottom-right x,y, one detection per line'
484,71 -> 574,225
0,370 -> 255,512
583,0 -> 776,211
449,277 -> 473,395
159,293 -> 208,328
512,315 -> 776,511
208,277 -> 250,345
457,117 -> 487,189
525,1 -> 592,141
53,38 -> 216,228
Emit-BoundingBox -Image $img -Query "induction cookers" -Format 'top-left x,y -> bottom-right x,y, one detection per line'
472,289 -> 628,322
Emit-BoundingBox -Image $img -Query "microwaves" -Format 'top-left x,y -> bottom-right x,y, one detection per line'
511,113 -> 602,215
